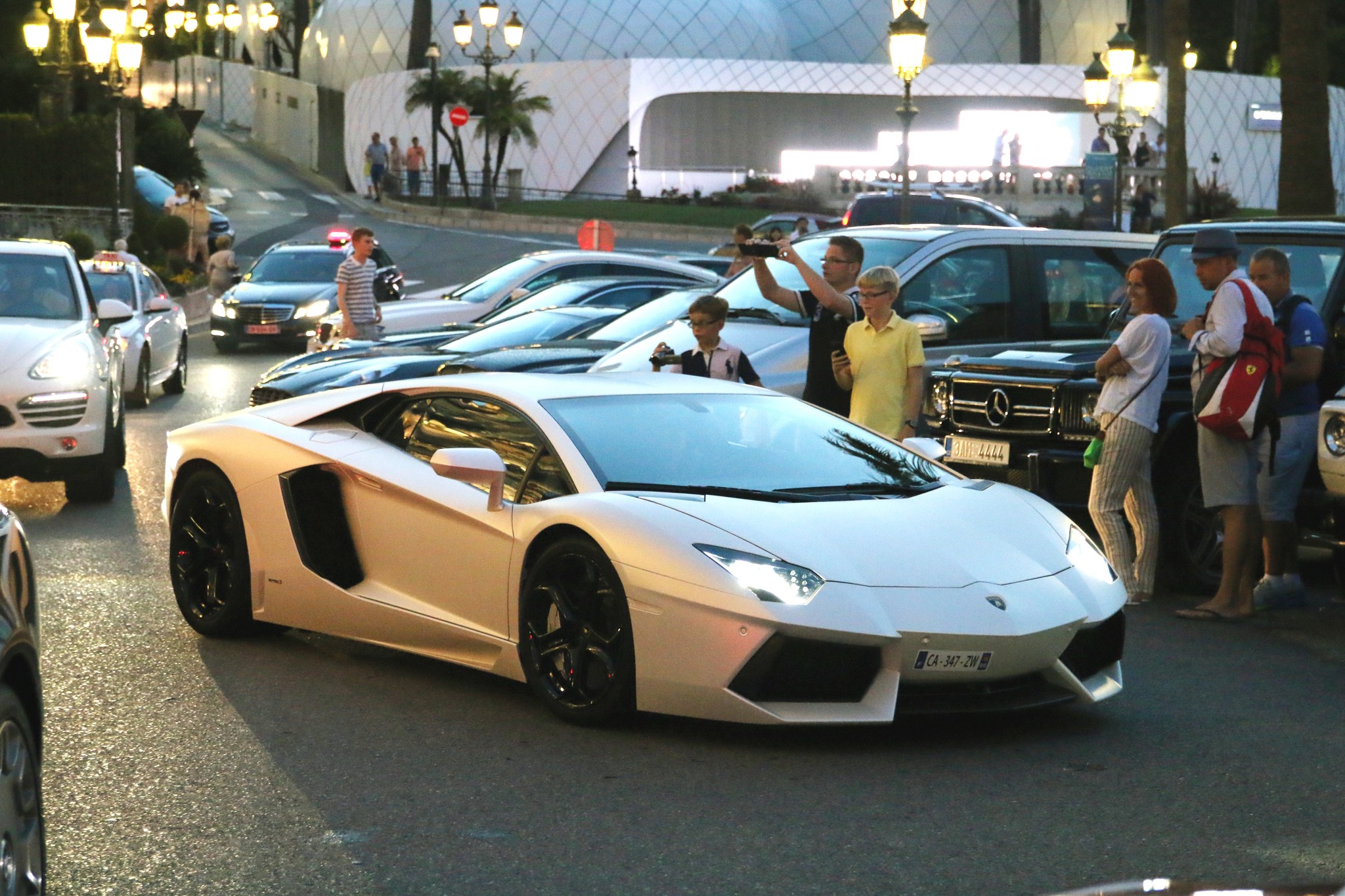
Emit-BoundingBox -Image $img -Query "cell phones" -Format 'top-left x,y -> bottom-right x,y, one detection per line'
737,242 -> 779,257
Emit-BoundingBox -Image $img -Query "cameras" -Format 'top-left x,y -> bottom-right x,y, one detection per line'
648,347 -> 682,367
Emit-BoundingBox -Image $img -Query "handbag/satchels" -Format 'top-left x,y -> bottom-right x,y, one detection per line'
1084,431 -> 1105,468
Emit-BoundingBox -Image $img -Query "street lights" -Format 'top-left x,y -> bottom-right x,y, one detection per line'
164,3 -> 244,131
453,0 -> 524,212
76,7 -> 143,247
885,0 -> 932,227
1083,19 -> 1163,237
425,42 -> 442,205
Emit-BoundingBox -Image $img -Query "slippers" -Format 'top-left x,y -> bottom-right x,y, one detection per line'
1175,605 -> 1254,622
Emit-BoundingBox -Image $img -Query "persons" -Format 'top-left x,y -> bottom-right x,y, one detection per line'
205,234 -> 238,303
1092,126 -> 1167,239
647,295 -> 766,390
831,264 -> 925,443
364,132 -> 427,204
742,236 -> 864,418
334,227 -> 383,339
113,239 -> 140,263
164,181 -> 211,266
722,217 -> 810,279
1174,228 -> 1274,623
1250,247 -> 1327,608
1088,258 -> 1178,605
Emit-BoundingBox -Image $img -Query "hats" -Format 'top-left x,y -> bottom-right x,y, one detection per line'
1186,229 -> 1242,261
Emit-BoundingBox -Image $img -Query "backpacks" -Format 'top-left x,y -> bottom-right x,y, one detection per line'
1193,279 -> 1285,440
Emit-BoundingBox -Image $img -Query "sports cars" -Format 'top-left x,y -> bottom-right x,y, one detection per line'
165,373 -> 1133,725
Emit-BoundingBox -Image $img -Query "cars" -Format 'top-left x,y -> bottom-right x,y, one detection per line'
42,250 -> 191,411
208,230 -> 408,353
839,187 -> 1069,286
251,275 -> 719,415
0,240 -> 128,507
304,249 -> 729,361
127,165 -> 236,259
0,505 -> 48,895
708,211 -> 844,273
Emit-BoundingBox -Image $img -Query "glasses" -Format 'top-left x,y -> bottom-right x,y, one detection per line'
820,258 -> 855,264
688,320 -> 721,329
856,291 -> 891,300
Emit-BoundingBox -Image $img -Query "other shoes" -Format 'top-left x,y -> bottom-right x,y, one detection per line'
1253,578 -> 1289,611
1282,579 -> 1308,606
1126,590 -> 1152,605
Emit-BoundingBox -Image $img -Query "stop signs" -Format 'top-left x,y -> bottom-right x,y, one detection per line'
448,107 -> 469,128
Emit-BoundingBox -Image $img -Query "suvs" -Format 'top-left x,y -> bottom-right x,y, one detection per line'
916,219 -> 1345,607
591,225 -> 1179,448
